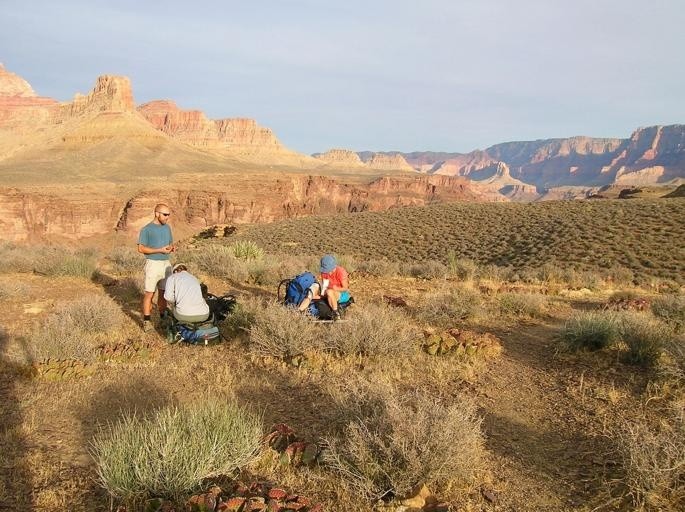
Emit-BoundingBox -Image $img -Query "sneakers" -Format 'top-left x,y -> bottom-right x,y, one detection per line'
144,320 -> 152,332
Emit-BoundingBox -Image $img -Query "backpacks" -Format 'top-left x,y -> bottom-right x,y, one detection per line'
278,271 -> 336,320
177,323 -> 221,345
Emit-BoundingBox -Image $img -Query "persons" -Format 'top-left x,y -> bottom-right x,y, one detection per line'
164,263 -> 210,324
298,256 -> 350,320
137,204 -> 175,323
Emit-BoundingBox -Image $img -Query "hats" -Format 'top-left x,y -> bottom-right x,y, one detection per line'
318,255 -> 337,273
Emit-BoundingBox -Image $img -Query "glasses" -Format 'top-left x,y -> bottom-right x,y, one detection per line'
155,210 -> 170,216
